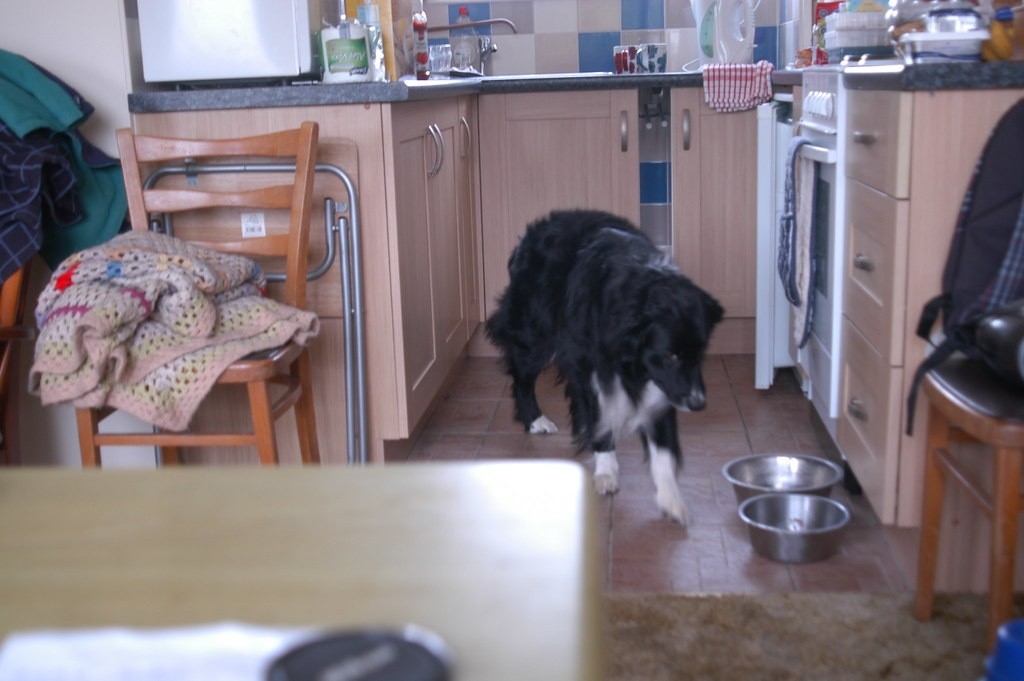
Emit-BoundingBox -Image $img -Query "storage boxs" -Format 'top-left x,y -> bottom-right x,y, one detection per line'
898,32 -> 990,65
822,12 -> 896,63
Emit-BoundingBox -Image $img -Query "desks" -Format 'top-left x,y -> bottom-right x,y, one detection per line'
1,455 -> 617,681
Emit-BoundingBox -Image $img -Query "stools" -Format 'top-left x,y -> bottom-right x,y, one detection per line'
913,360 -> 1024,651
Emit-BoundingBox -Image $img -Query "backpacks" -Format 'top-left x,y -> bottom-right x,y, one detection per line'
916,97 -> 1023,379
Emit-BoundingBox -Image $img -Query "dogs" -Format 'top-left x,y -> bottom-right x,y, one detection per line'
484,206 -> 727,528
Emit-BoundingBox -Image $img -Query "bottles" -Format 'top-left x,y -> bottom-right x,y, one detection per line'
450,7 -> 481,73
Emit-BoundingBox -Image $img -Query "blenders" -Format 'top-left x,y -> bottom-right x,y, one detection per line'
689,1 -> 757,66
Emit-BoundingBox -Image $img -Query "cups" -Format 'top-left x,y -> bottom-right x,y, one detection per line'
429,44 -> 452,80
613,42 -> 668,74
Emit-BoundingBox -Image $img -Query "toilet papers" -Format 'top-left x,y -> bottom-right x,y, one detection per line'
316,24 -> 376,85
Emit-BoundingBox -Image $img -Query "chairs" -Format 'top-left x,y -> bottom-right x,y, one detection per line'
74,120 -> 320,466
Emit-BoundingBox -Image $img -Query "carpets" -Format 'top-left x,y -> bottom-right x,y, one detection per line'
604,590 -> 1024,681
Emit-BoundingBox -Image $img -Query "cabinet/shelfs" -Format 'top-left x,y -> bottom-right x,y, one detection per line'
132,87 -> 1024,530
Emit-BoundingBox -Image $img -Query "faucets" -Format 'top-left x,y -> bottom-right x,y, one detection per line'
478,37 -> 498,74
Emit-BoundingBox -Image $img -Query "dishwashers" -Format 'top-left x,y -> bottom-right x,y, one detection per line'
790,121 -> 845,421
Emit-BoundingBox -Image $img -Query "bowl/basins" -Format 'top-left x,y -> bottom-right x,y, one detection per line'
899,30 -> 991,64
737,492 -> 850,563
721,453 -> 844,504
